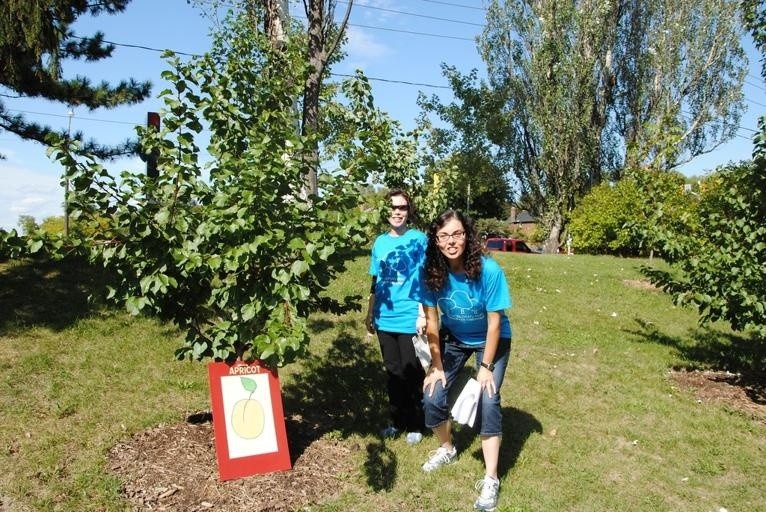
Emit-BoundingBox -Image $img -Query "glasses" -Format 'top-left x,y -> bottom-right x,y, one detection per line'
392,203 -> 408,212
435,231 -> 467,242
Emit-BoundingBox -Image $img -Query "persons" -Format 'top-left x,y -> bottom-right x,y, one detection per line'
416,205 -> 512,511
362,188 -> 431,447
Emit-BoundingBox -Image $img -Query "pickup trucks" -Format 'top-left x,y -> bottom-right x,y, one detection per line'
481,237 -> 532,253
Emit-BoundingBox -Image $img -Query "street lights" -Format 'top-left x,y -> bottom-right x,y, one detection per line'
60,107 -> 74,241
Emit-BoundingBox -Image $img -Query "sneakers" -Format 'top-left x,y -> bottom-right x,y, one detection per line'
380,428 -> 397,438
424,444 -> 457,472
407,432 -> 422,446
476,478 -> 500,510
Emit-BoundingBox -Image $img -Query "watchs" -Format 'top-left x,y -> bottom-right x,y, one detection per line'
480,361 -> 495,371
